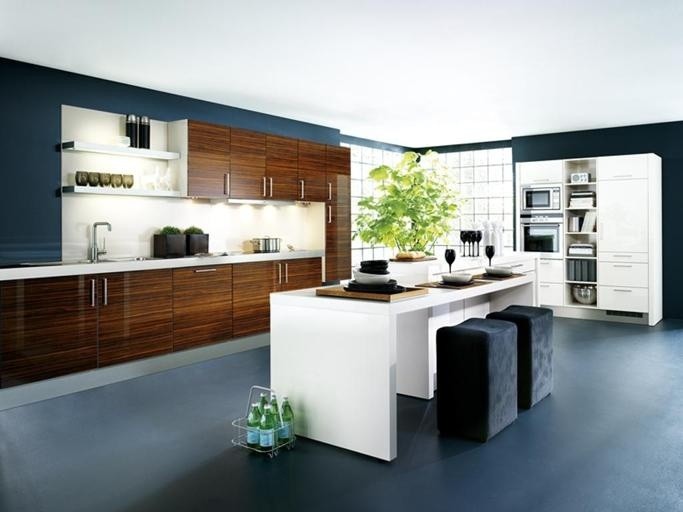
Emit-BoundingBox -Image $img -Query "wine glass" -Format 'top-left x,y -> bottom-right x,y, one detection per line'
444,249 -> 456,273
460,229 -> 482,257
486,245 -> 494,266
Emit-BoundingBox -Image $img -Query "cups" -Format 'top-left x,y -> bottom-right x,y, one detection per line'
75,170 -> 133,189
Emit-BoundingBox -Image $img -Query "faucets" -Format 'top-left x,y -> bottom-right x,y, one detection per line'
91,221 -> 111,260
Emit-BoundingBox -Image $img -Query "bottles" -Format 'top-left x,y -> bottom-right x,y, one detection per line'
126,114 -> 138,147
139,115 -> 150,149
246,393 -> 295,451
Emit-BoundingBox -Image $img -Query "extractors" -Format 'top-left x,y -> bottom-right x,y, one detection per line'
210,199 -> 296,207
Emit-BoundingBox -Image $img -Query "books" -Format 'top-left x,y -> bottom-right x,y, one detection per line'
568,212 -> 597,232
569,190 -> 596,208
568,259 -> 597,282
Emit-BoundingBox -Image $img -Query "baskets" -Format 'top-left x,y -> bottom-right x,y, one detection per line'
232,386 -> 297,459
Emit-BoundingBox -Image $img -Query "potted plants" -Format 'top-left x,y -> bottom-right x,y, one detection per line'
350,150 -> 469,256
184,226 -> 208,256
154,225 -> 186,259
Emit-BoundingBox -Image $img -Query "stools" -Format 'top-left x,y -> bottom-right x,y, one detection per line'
486,304 -> 553,408
436,317 -> 518,443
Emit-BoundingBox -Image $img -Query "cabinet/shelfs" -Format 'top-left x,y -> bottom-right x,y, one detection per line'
229,127 -> 298,201
597,153 -> 663,314
297,140 -> 327,203
174,264 -> 233,354
168,119 -> 230,199
233,257 -> 322,341
563,157 -> 597,309
0,269 -> 173,390
515,160 -> 562,306
326,145 -> 352,281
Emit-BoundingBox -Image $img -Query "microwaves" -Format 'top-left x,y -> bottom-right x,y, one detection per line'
520,179 -> 563,215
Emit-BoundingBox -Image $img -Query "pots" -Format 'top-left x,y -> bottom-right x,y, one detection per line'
249,237 -> 282,252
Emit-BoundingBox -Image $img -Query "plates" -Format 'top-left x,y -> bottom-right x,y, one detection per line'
343,285 -> 407,293
485,267 -> 512,275
349,280 -> 397,289
442,274 -> 473,283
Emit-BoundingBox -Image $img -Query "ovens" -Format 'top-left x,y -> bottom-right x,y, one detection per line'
520,215 -> 564,259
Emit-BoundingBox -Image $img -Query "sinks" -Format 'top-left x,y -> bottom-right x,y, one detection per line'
79,256 -> 155,264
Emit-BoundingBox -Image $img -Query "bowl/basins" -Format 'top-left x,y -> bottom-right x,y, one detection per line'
353,259 -> 390,285
573,285 -> 597,305
